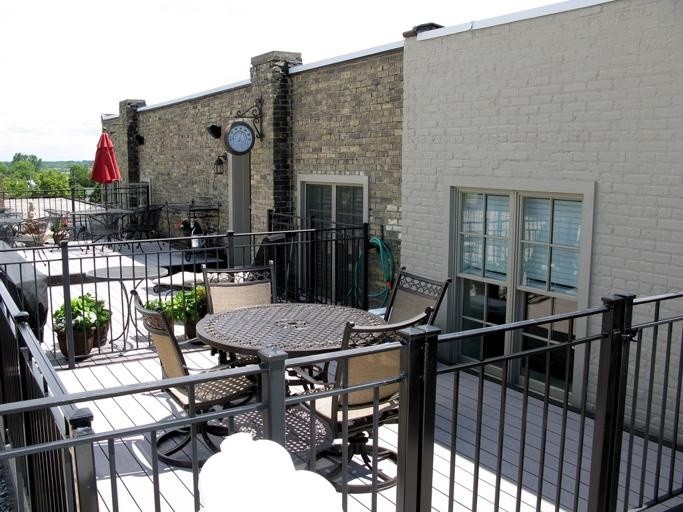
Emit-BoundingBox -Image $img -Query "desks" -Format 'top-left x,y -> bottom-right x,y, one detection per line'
196,303 -> 390,406
86,264 -> 170,357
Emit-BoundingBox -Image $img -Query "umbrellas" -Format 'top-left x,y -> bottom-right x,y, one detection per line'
91,127 -> 122,211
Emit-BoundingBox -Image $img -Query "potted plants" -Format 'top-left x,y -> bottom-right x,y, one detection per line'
50,291 -> 112,363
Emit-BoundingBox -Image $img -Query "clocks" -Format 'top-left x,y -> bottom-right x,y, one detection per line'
223,96 -> 265,157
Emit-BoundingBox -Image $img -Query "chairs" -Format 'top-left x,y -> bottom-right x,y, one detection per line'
383,266 -> 453,341
126,287 -> 262,469
202,259 -> 276,369
293,306 -> 435,492
0,201 -> 166,267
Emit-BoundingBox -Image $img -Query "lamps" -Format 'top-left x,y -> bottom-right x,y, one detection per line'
213,152 -> 227,175
